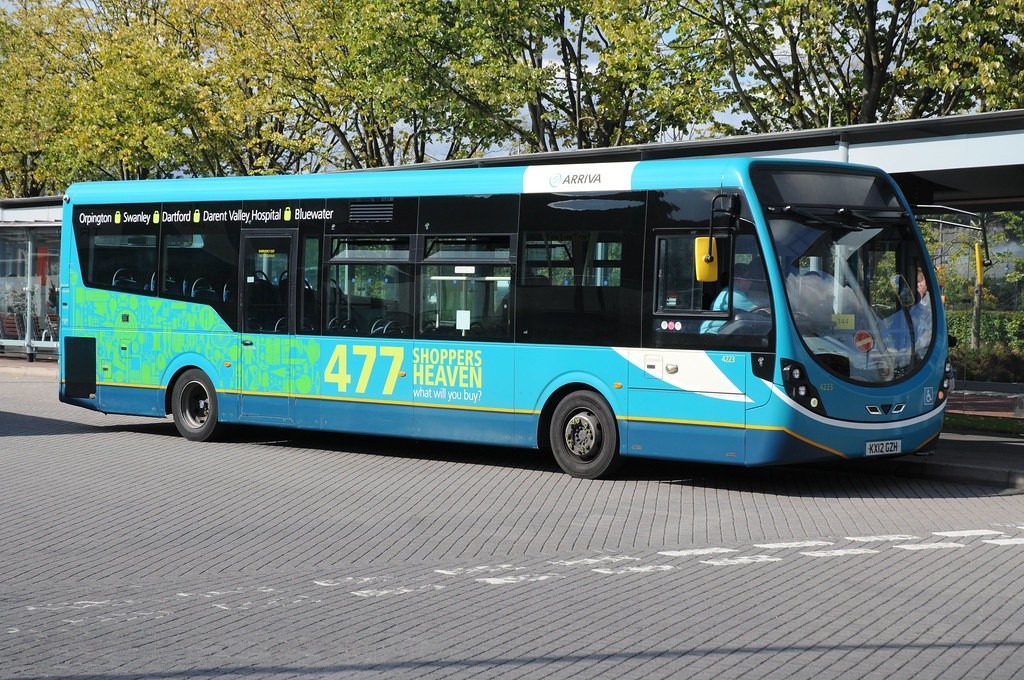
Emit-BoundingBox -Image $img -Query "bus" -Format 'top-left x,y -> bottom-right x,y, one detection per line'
57,151 -> 954,480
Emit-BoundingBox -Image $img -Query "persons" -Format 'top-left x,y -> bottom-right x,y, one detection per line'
700,263 -> 770,335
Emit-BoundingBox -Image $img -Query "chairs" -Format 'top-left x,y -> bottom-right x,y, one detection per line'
109,267 -> 504,340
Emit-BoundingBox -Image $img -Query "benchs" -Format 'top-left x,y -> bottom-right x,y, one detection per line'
44,314 -> 59,342
0,313 -> 50,363
